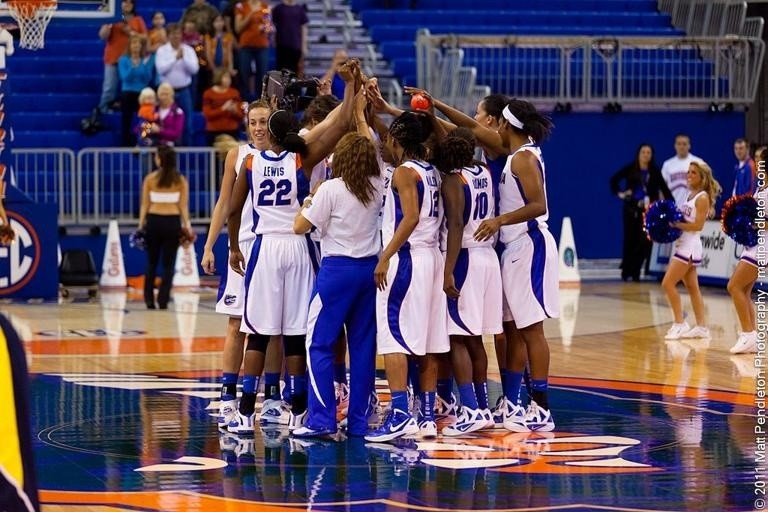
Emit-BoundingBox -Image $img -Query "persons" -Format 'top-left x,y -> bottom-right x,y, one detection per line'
727,145 -> 768,354
728,354 -> 768,512
660,132 -> 706,204
661,162 -> 723,340
609,143 -> 675,281
729,138 -> 763,197
662,338 -> 708,508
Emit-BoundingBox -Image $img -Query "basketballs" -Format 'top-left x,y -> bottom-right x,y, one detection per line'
411,94 -> 429,109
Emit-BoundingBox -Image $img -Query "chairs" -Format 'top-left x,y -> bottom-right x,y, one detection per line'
56,249 -> 99,303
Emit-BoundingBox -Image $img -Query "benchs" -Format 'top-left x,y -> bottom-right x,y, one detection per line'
0,0 -> 308,229
342,0 -> 729,106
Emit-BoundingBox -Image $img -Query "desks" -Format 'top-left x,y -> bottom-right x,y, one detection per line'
646,218 -> 768,293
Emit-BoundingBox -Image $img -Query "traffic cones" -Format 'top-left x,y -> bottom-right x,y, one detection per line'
172,237 -> 202,289
555,217 -> 583,283
174,293 -> 203,360
100,291 -> 128,362
555,287 -> 584,352
96,216 -> 128,288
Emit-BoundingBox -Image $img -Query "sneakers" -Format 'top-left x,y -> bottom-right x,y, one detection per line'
432,391 -> 459,423
662,319 -> 693,340
233,436 -> 257,458
218,433 -> 239,453
680,324 -> 711,342
337,391 -> 381,430
730,356 -> 761,378
401,407 -> 438,440
682,339 -> 712,352
261,423 -> 288,449
292,424 -> 339,437
331,380 -> 355,422
217,400 -> 240,427
288,409 -> 310,432
440,406 -> 488,437
729,329 -> 758,355
260,399 -> 295,426
226,409 -> 257,435
364,407 -> 420,444
663,340 -> 691,357
486,396 -> 529,430
475,407 -> 497,430
286,436 -> 317,454
503,401 -> 557,434
747,335 -> 765,354
361,442 -> 422,470
